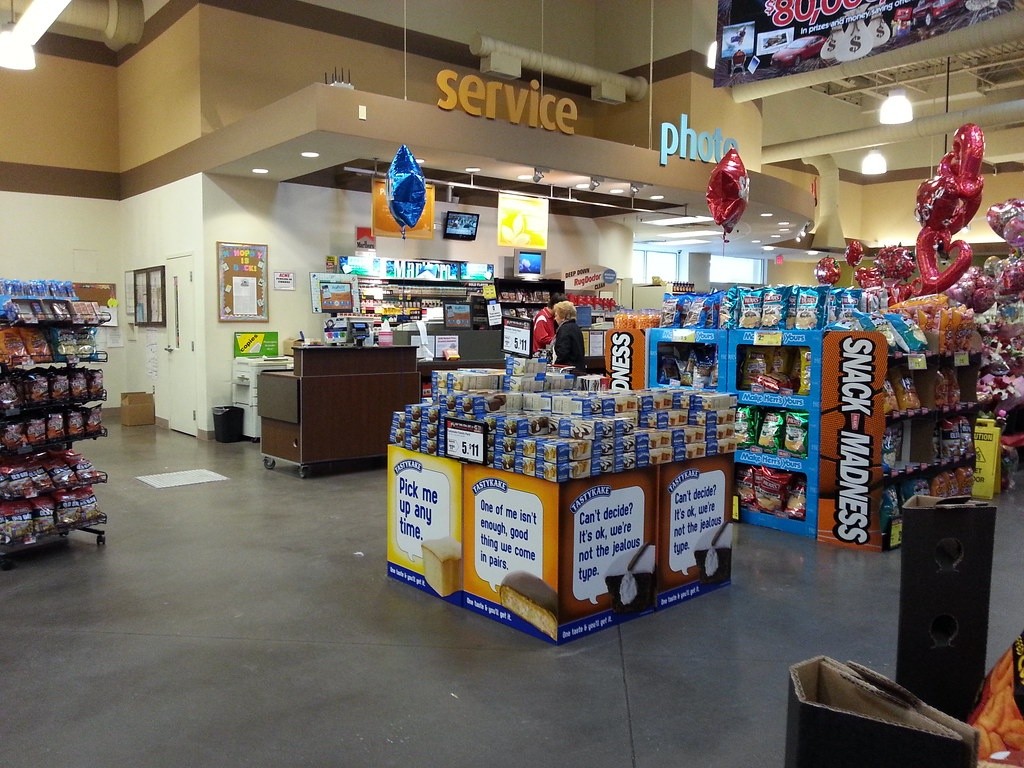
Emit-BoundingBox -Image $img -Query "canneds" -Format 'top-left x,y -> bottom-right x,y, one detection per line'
404,302 -> 420,308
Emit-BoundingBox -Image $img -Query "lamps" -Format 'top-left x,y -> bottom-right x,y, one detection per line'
629,183 -> 643,197
531,167 -> 549,183
860,145 -> 888,175
878,68 -> 913,125
588,175 -> 603,191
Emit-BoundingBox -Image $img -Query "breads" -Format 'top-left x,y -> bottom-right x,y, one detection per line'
421,537 -> 462,596
499,571 -> 557,641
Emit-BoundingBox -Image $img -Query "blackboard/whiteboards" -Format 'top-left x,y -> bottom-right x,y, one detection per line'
309,271 -> 361,315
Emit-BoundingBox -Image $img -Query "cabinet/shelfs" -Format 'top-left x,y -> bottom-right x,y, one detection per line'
0,308 -> 108,569
336,275 -> 567,331
640,327 -> 986,553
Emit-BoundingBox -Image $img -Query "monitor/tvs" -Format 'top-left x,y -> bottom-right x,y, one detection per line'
443,211 -> 480,241
346,317 -> 374,343
513,248 -> 546,281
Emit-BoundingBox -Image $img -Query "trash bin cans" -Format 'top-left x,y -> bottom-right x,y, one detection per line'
211,404 -> 245,443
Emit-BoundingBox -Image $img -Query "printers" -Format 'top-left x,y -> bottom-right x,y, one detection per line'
233,356 -> 293,437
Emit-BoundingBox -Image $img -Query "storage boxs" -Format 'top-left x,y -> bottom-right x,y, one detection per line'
390,358 -> 736,481
120,392 -> 155,427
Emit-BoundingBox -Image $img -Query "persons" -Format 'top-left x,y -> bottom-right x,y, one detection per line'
533,293 -> 569,354
550,301 -> 585,373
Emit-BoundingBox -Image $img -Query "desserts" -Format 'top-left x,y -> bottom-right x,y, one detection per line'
694,522 -> 733,581
605,542 -> 655,613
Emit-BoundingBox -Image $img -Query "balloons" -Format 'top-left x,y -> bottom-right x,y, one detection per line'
385,144 -> 426,238
845,240 -> 864,266
706,147 -> 750,242
855,122 -> 1024,311
814,257 -> 842,285
